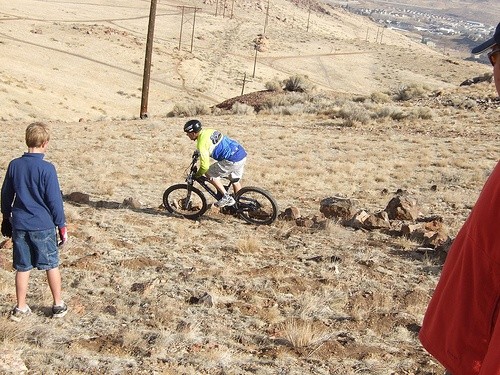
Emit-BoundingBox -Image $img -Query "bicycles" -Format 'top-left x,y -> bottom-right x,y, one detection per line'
162,150 -> 278,226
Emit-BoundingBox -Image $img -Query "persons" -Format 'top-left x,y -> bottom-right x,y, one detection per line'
184,119 -> 247,209
0,121 -> 69,322
418,23 -> 500,375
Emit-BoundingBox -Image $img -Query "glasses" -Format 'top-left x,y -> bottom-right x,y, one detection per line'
487,49 -> 500,66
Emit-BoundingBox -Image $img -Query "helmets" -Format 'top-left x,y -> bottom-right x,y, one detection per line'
184,120 -> 201,133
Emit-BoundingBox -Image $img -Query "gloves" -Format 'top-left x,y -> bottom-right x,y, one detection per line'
188,174 -> 196,181
192,150 -> 199,158
1,218 -> 12,238
58,227 -> 68,246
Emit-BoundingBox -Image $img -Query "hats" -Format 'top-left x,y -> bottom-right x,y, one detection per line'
472,23 -> 500,55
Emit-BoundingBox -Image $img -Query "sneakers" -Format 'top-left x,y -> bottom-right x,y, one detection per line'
52,300 -> 68,317
10,303 -> 32,321
214,195 -> 236,207
